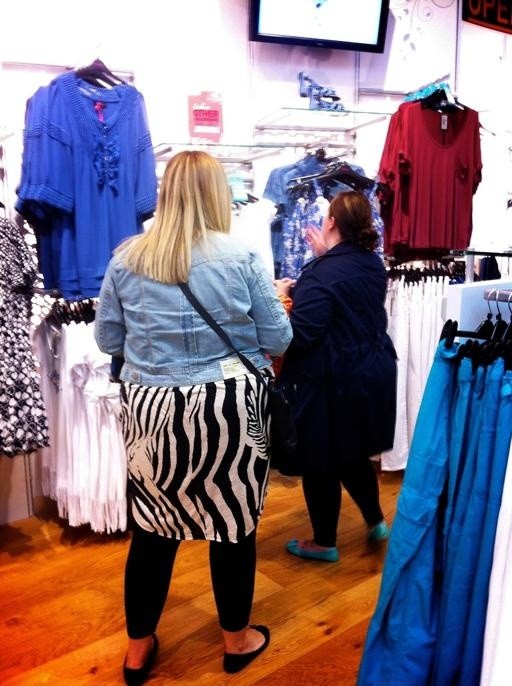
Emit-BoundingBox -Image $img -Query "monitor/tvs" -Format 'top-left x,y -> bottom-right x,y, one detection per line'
249,0 -> 390,53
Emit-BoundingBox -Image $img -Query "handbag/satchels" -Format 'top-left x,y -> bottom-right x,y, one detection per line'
266,379 -> 314,459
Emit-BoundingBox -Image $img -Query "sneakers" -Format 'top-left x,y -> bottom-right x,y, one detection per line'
223,624 -> 270,674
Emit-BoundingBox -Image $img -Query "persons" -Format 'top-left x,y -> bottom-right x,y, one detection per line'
273,190 -> 397,563
94,149 -> 294,686
0,206 -> 50,461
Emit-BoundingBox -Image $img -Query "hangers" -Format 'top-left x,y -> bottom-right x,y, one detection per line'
285,141 -> 375,197
388,255 -> 512,375
45,299 -> 97,333
75,43 -> 128,88
403,80 -> 465,111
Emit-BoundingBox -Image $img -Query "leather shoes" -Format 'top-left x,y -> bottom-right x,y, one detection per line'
123,632 -> 158,686
367,522 -> 390,551
287,540 -> 339,562
